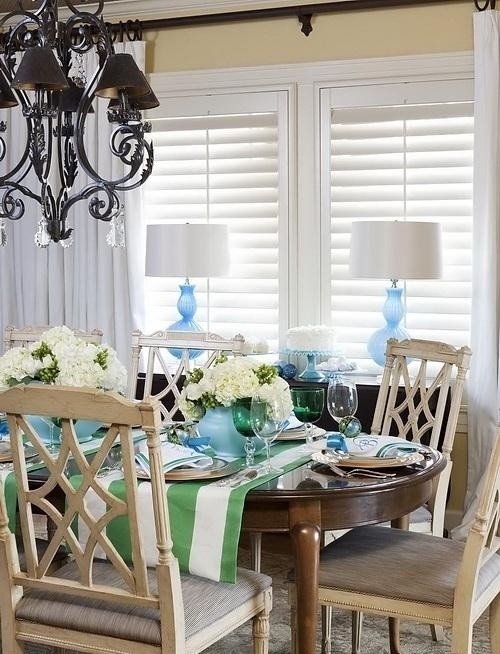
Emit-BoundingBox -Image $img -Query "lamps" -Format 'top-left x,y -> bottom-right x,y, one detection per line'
1,0 -> 161,251
140,221 -> 230,361
347,218 -> 444,370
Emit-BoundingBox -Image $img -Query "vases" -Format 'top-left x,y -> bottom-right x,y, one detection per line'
196,405 -> 282,456
21,415 -> 106,445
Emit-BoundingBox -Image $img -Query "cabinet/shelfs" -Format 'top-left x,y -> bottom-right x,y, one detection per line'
135,370 -> 454,536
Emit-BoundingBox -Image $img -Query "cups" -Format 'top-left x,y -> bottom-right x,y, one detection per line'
326,377 -> 360,427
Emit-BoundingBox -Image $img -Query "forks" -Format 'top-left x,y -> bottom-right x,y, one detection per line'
328,463 -> 396,480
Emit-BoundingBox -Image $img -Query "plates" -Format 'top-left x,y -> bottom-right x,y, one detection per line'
120,457 -> 240,481
271,423 -> 326,443
0,441 -> 39,464
312,451 -> 428,468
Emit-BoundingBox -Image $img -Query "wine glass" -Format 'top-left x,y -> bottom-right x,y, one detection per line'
40,416 -> 57,452
290,387 -> 327,455
248,392 -> 288,476
229,396 -> 265,474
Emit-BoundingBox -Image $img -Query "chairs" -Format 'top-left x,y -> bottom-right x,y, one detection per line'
288,425 -> 500,652
128,328 -> 246,423
1,322 -> 103,352
0,385 -> 276,653
319,336 -> 473,653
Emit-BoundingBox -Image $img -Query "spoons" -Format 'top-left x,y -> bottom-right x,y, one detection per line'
230,470 -> 259,489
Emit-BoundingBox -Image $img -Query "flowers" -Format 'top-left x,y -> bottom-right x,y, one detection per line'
1,324 -> 126,398
176,357 -> 297,427
